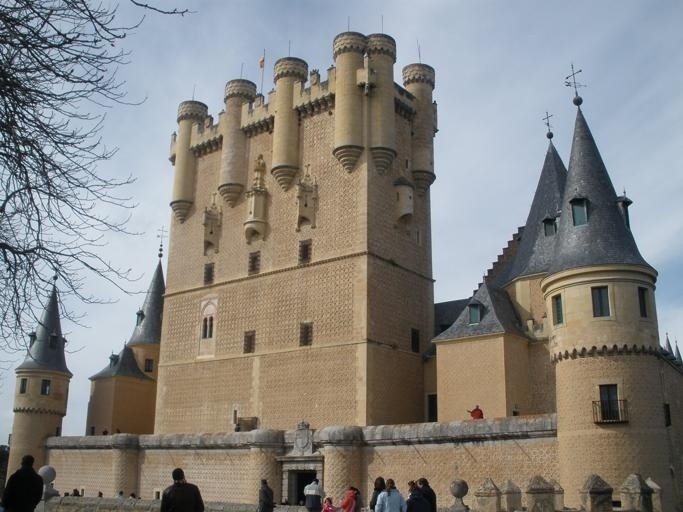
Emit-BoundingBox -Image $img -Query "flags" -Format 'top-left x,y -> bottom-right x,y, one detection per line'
258,54 -> 264,68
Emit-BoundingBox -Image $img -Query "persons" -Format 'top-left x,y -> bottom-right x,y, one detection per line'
354,487 -> 361,511
470,405 -> 482,419
373,479 -> 407,512
158,467 -> 204,512
404,480 -> 431,512
369,477 -> 385,512
339,486 -> 355,512
0,455 -> 44,511
303,477 -> 325,512
65,486 -> 135,498
512,404 -> 520,416
257,478 -> 274,511
321,497 -> 336,512
416,477 -> 435,512
